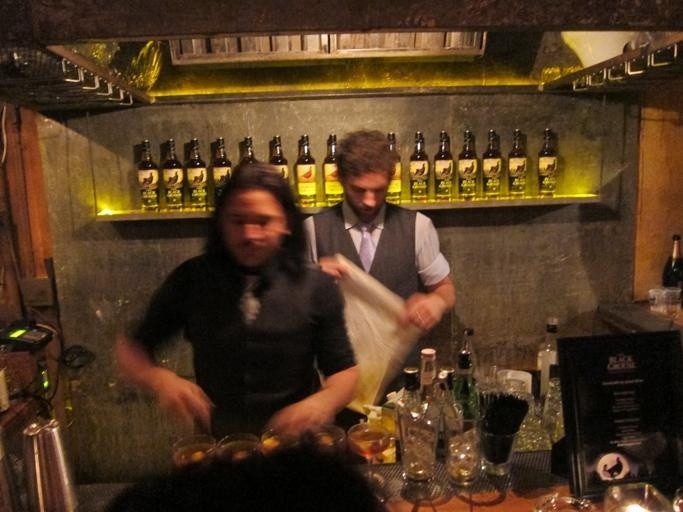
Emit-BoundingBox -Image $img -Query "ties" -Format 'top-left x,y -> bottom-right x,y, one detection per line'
356,223 -> 376,274
238,275 -> 263,324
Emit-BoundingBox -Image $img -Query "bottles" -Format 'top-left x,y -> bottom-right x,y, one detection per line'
480,127 -> 504,198
532,316 -> 561,413
182,134 -> 209,209
402,326 -> 482,416
384,131 -> 406,205
408,131 -> 431,203
267,134 -> 291,181
506,127 -> 529,197
236,134 -> 257,163
663,234 -> 682,300
535,127 -> 560,196
293,133 -> 319,208
210,135 -> 235,207
131,138 -> 160,214
156,139 -> 186,211
458,129 -> 479,199
321,132 -> 345,208
433,129 -> 456,201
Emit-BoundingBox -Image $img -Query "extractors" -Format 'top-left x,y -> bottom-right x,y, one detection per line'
0,0 -> 682,125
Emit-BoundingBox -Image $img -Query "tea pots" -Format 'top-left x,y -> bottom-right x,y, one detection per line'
24,417 -> 78,512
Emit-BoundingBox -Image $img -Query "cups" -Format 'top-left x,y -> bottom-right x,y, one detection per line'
172,422 -> 344,474
443,418 -> 483,485
540,377 -> 564,447
394,410 -> 438,492
504,390 -> 534,449
479,389 -> 520,478
528,485 -> 683,512
647,288 -> 680,317
498,369 -> 531,395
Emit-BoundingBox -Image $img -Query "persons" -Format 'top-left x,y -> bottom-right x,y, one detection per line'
113,163 -> 358,447
300,130 -> 457,433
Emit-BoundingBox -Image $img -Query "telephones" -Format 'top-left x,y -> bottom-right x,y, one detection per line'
1,320 -> 56,350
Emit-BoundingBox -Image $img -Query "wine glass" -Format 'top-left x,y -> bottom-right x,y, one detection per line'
349,423 -> 389,489
